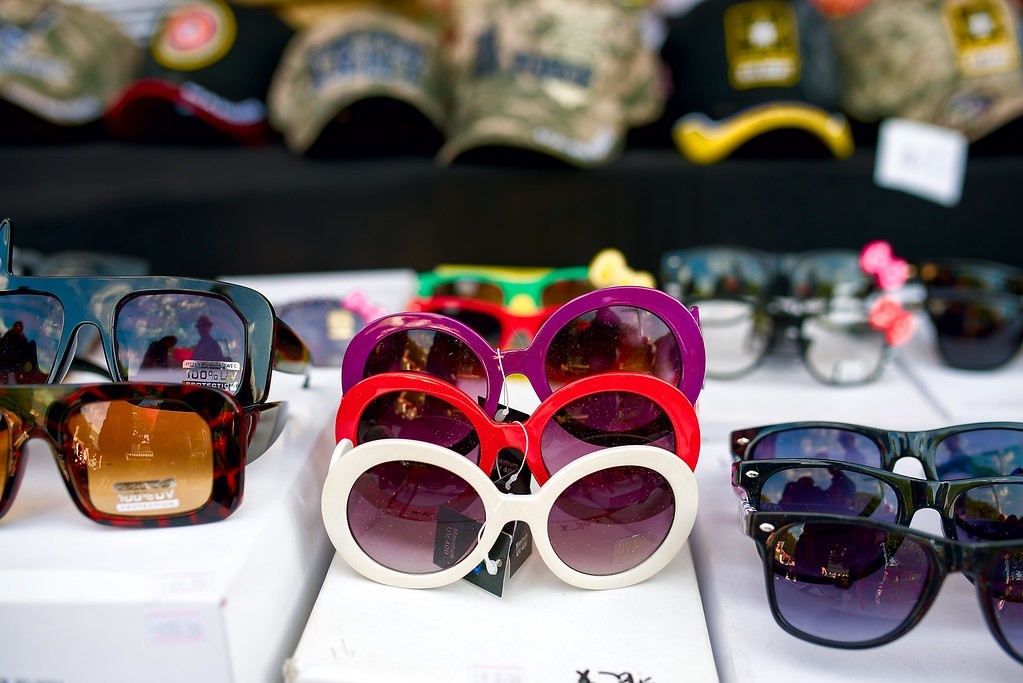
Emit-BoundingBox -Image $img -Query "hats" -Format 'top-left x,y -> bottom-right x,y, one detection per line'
435,0 -> 658,170
102,1 -> 302,137
1,0 -> 138,123
841,1 -> 1023,143
659,0 -> 855,161
195,315 -> 215,328
267,16 -> 450,153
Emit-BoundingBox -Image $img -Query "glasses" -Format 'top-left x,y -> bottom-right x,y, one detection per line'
0,219 -> 314,420
280,250 -> 707,447
729,420 -> 1023,663
336,373 -> 702,525
0,383 -> 280,529
679,247 -> 1022,389
322,438 -> 698,590
